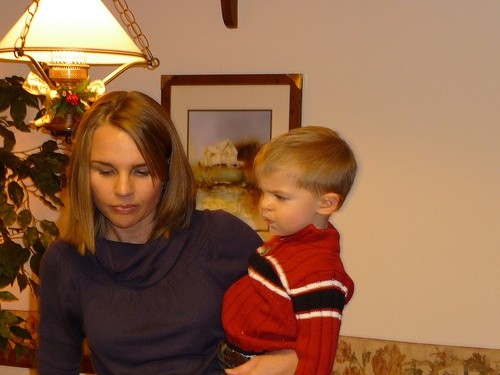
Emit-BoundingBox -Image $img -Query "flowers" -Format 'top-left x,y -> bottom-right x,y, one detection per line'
50,79 -> 95,116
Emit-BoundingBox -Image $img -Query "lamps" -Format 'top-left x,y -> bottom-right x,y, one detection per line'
0,1 -> 160,145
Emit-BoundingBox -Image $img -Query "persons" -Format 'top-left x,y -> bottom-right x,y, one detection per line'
36,90 -> 299,375
217,126 -> 358,375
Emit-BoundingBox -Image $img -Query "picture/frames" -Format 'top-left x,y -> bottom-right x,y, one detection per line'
161,74 -> 303,243
